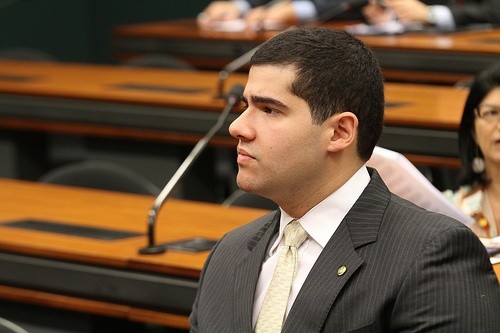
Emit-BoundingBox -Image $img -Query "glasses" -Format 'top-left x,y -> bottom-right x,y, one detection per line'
474,104 -> 500,122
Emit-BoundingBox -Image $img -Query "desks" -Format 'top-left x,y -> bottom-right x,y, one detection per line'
0,22 -> 500,333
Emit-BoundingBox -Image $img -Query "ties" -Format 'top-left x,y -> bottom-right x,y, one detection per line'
254,220 -> 309,333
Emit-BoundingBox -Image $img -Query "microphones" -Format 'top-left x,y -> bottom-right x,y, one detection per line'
138,84 -> 244,254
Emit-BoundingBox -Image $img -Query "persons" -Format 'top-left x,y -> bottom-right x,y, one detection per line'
189,28 -> 500,333
197,0 -> 500,34
443,69 -> 500,238
